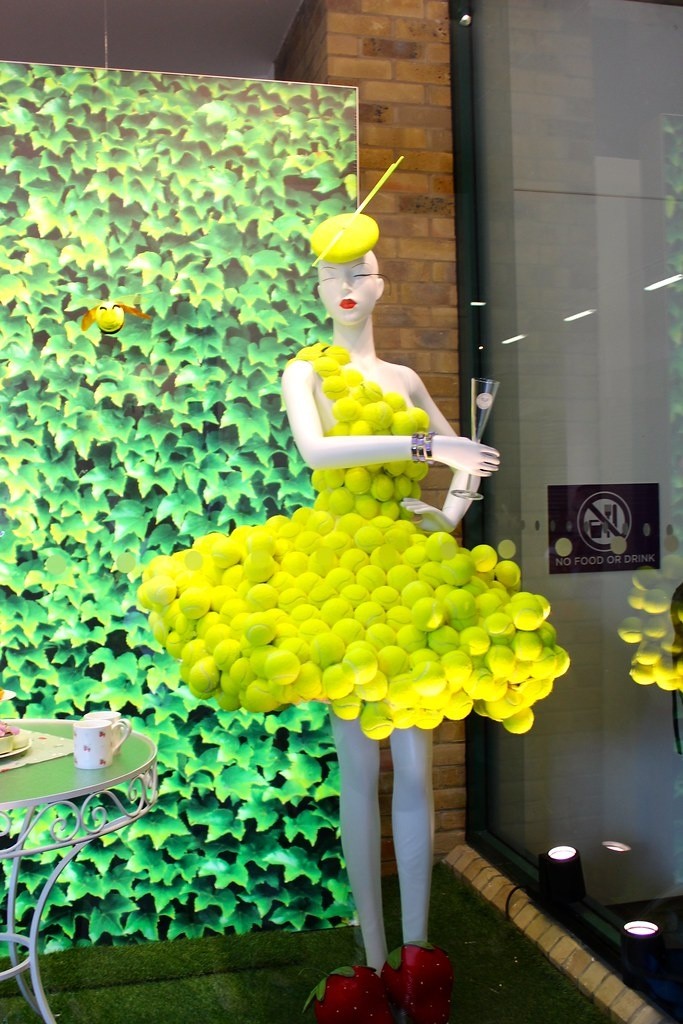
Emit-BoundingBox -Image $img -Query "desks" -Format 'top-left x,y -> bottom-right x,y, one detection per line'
0,719 -> 157,1024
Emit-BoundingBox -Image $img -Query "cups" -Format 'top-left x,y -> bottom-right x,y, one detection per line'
84,710 -> 132,757
75,719 -> 127,769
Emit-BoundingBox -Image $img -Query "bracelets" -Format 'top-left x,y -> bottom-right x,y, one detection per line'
411,431 -> 438,465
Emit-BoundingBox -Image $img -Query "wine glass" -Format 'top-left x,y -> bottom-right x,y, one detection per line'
452,377 -> 500,501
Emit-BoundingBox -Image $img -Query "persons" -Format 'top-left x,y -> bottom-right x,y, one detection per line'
279,212 -> 500,977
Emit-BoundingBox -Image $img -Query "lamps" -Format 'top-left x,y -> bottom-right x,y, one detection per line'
621,920 -> 660,989
538,846 -> 587,908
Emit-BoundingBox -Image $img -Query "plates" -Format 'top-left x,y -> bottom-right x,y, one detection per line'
0,738 -> 31,758
0,690 -> 16,701
